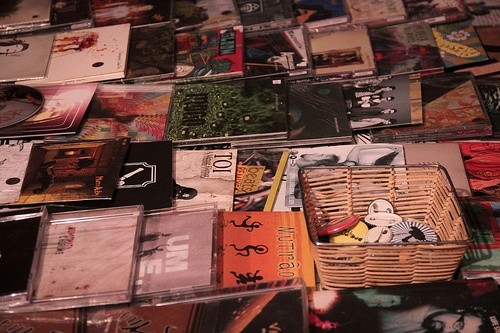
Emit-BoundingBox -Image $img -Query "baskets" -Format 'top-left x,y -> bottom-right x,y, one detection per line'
298,165 -> 472,290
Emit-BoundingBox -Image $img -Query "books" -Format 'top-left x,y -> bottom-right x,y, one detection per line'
0,0 -> 500,333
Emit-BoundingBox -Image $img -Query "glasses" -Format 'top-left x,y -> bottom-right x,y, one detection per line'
452,312 -> 466,333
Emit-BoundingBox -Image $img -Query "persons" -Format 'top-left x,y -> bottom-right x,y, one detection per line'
294,153 -> 339,168
345,106 -> 397,117
173,181 -> 197,200
347,116 -> 397,128
343,86 -> 396,98
307,288 -> 496,333
343,96 -> 394,108
